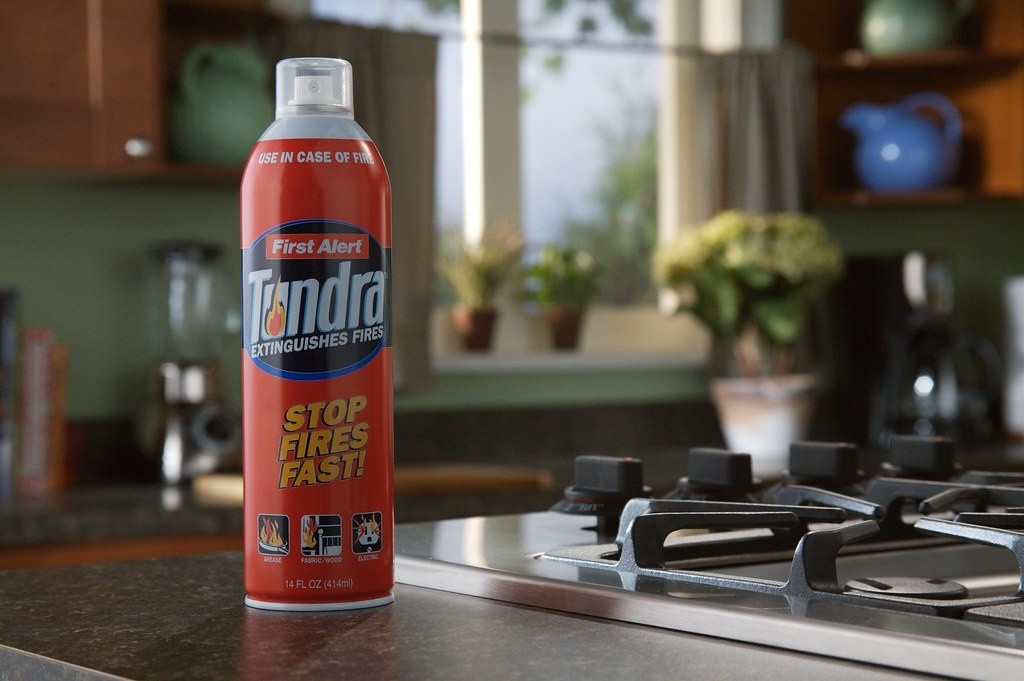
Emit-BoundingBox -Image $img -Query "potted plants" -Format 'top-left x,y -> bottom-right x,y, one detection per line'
656,210 -> 844,480
525,245 -> 596,353
437,236 -> 525,351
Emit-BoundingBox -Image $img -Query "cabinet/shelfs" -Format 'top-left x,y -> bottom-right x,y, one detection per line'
0,0 -> 291,189
783,0 -> 1024,210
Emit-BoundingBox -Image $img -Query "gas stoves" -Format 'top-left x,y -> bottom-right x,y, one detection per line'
388,435 -> 1024,681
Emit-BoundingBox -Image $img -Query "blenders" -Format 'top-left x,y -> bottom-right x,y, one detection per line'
147,236 -> 240,481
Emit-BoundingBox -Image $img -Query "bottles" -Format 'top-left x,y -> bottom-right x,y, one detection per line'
17,331 -> 63,493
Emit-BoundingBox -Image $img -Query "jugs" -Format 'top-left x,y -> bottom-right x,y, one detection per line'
842,95 -> 962,184
176,46 -> 270,164
241,56 -> 393,612
